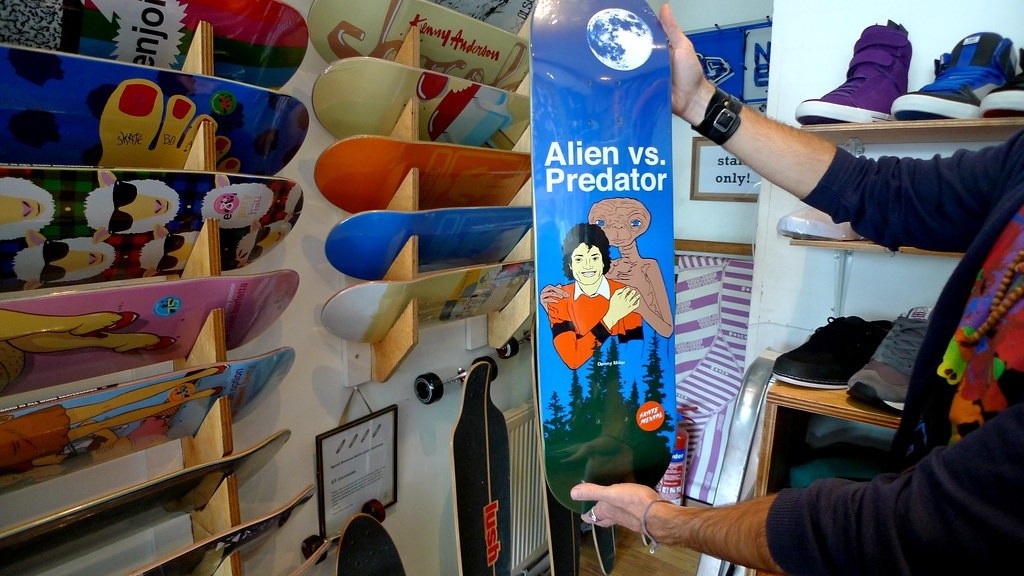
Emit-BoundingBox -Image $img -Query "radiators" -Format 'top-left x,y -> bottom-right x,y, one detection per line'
503,400 -> 548,576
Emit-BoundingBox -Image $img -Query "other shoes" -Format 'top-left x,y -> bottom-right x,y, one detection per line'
889,32 -> 1019,119
771,314 -> 894,390
795,19 -> 912,123
979,48 -> 1024,117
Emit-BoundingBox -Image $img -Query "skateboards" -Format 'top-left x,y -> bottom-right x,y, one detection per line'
495,309 -> 582,576
412,355 -> 512,576
1,0 -> 338,576
527,1 -> 681,516
641,533 -> 652,546
305,1 -> 540,346
299,497 -> 408,576
591,523 -> 618,576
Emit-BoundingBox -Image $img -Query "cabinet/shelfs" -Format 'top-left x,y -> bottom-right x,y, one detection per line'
788,116 -> 1023,258
744,382 -> 901,576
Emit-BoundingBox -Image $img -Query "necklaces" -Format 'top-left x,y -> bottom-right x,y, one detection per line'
970,251 -> 1023,342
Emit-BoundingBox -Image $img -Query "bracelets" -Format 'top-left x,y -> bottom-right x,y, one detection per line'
642,500 -> 672,552
692,88 -> 742,145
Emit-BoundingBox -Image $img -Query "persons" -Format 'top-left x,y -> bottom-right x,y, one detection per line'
570,4 -> 1024,576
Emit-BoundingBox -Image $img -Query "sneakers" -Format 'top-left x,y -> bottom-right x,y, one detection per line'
846,307 -> 932,414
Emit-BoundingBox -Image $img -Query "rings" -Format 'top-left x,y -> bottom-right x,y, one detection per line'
589,508 -> 598,522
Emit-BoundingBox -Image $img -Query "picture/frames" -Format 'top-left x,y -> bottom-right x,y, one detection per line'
690,137 -> 762,204
